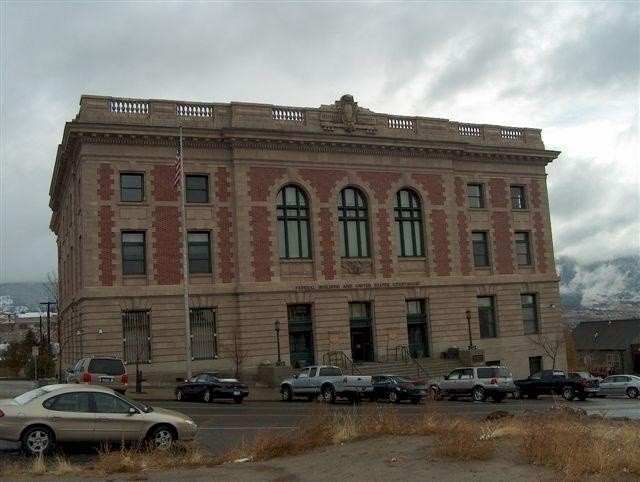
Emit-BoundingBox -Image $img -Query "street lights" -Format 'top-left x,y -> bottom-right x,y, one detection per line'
465,309 -> 477,350
275,320 -> 285,366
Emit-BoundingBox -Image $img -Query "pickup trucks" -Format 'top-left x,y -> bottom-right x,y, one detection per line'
280,365 -> 374,403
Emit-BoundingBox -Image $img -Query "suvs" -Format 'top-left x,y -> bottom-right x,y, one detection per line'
429,366 -> 516,402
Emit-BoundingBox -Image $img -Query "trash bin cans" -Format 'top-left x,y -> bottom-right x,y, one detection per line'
448,348 -> 458,360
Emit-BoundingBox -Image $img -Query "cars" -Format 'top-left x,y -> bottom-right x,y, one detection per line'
66,356 -> 129,393
512,370 -> 640,400
175,372 -> 249,403
0,383 -> 198,451
360,374 -> 428,403
